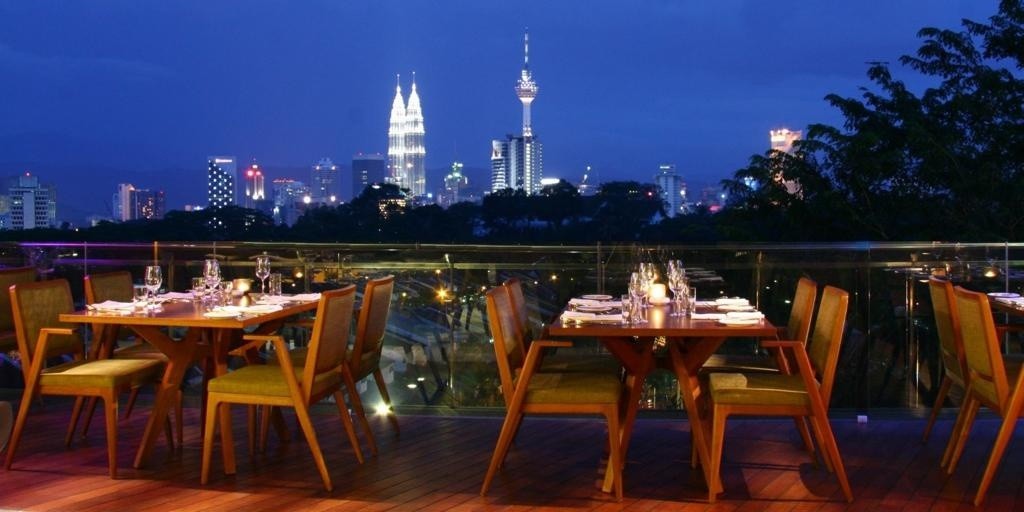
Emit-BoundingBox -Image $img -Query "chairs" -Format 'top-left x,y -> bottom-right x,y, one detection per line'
258,271 -> 396,455
691,274 -> 819,460
707,287 -> 855,502
8,277 -> 170,478
926,274 -> 1024,446
501,277 -> 628,467
944,283 -> 1024,508
201,284 -> 364,491
79,269 -> 211,443
481,286 -> 627,504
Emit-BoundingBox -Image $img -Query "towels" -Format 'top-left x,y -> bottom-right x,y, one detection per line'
223,303 -> 283,314
994,295 -> 1024,305
94,299 -> 134,311
158,290 -> 192,299
563,310 -> 596,319
727,310 -> 765,320
716,298 -> 749,306
569,297 -> 599,306
290,292 -> 320,301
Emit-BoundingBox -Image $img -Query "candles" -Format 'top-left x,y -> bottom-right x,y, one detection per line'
650,283 -> 670,305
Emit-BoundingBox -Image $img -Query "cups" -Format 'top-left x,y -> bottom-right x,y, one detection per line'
218,281 -> 233,307
621,294 -> 639,325
638,262 -> 655,308
144,266 -> 162,313
629,272 -> 648,323
666,259 -> 696,317
268,273 -> 281,297
201,258 -> 222,301
255,257 -> 270,300
132,284 -> 148,314
191,278 -> 204,300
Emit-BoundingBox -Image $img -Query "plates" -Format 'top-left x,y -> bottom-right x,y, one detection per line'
201,312 -> 240,320
718,319 -> 760,327
716,305 -> 754,311
986,292 -> 1021,298
581,295 -> 612,299
575,305 -> 612,311
